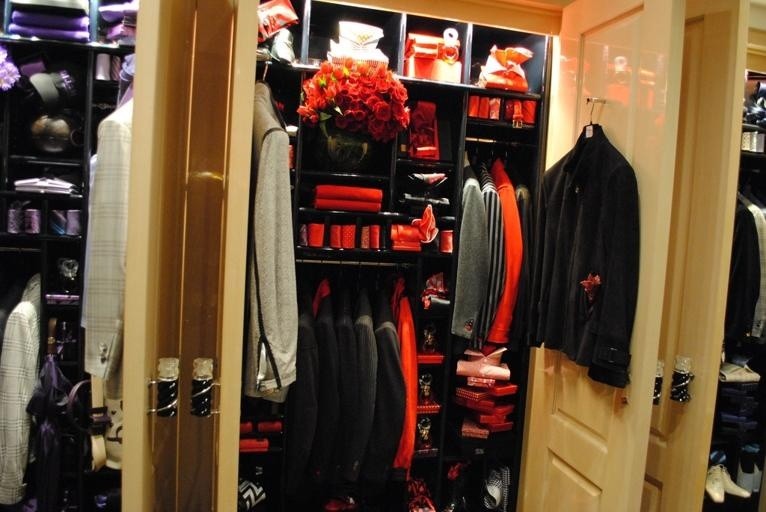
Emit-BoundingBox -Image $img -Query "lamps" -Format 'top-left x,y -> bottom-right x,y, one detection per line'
254,58 -> 273,87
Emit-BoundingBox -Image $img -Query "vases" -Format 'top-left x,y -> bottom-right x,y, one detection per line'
326,131 -> 369,172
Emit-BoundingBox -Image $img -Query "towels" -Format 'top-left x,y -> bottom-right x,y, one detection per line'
411,204 -> 439,244
388,222 -> 423,252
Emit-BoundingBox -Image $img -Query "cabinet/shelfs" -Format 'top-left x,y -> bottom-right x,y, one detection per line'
0,0 -> 139,512
240,0 -> 553,512
700,34 -> 766,512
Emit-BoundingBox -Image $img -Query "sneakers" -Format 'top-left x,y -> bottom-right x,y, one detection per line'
721,464 -> 753,500
704,464 -> 727,504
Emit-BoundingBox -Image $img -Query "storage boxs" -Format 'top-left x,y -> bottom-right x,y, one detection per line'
327,19 -> 385,49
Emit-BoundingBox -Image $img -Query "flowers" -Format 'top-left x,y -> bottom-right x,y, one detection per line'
296,58 -> 412,145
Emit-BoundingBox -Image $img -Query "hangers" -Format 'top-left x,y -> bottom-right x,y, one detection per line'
464,131 -> 512,167
579,96 -> 598,145
293,253 -> 402,298
